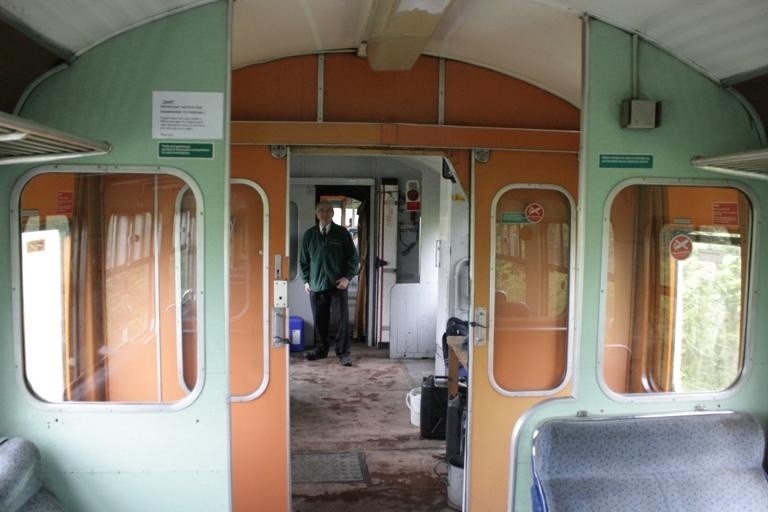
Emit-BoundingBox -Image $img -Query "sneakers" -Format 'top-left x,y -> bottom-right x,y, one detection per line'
304,348 -> 327,361
340,355 -> 353,366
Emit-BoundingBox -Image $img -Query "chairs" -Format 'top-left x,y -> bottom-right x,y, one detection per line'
528,410 -> 767,512
0,433 -> 75,512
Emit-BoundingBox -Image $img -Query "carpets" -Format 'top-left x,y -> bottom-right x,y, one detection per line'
290,450 -> 371,484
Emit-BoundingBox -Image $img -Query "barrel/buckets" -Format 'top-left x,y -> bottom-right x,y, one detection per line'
444,456 -> 465,512
405,387 -> 422,427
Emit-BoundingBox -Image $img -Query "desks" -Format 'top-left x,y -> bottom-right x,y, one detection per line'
446,335 -> 469,463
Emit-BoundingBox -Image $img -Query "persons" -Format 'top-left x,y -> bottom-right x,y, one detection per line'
298,199 -> 360,367
